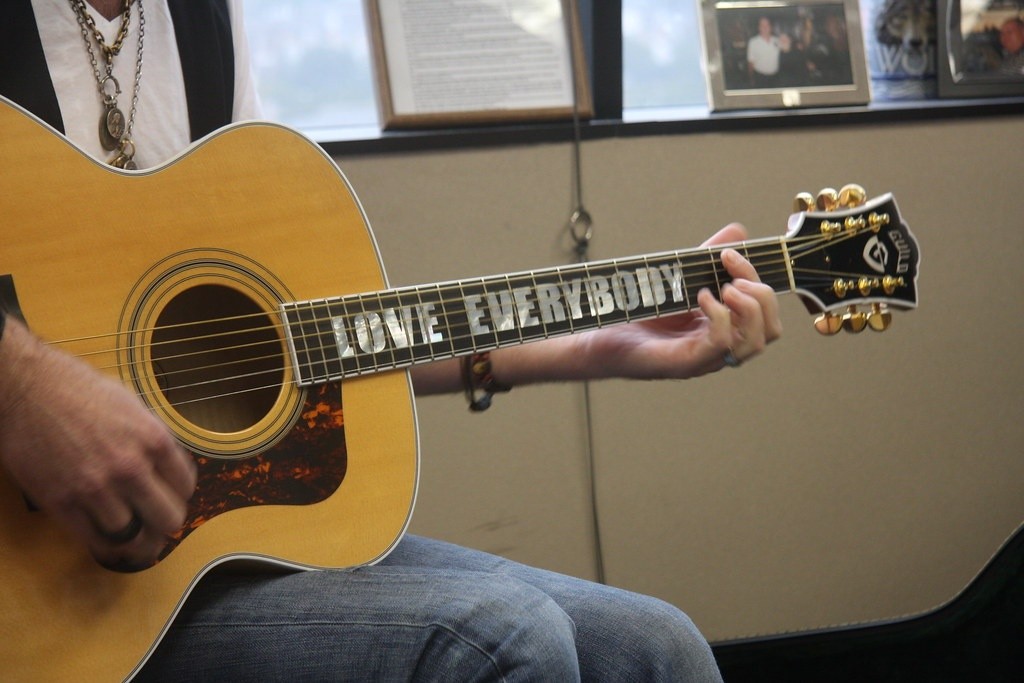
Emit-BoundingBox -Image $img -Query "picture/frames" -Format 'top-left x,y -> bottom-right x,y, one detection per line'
700,0 -> 872,113
936,0 -> 1024,98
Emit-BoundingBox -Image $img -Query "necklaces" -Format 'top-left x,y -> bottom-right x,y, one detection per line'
68,0 -> 145,170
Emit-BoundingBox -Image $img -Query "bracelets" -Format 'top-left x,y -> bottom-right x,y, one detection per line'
459,351 -> 513,412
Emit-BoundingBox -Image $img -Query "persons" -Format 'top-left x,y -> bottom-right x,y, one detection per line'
999,16 -> 1024,75
0,0 -> 783,683
725,11 -> 852,89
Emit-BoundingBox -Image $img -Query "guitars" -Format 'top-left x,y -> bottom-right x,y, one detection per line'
0,92 -> 920,683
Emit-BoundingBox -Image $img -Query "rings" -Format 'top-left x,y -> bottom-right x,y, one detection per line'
723,355 -> 741,368
93,507 -> 145,543
